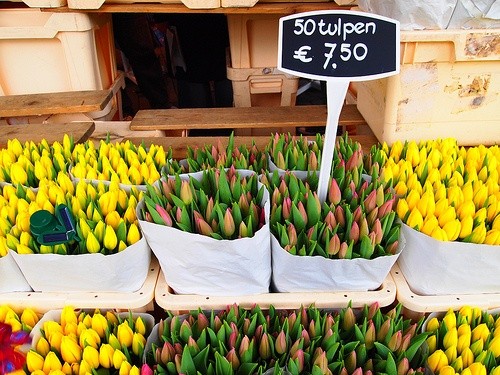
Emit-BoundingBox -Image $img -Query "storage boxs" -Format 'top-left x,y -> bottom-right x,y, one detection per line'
0,0 -> 500,148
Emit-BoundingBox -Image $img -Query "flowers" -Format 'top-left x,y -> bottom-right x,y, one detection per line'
0,131 -> 500,375
